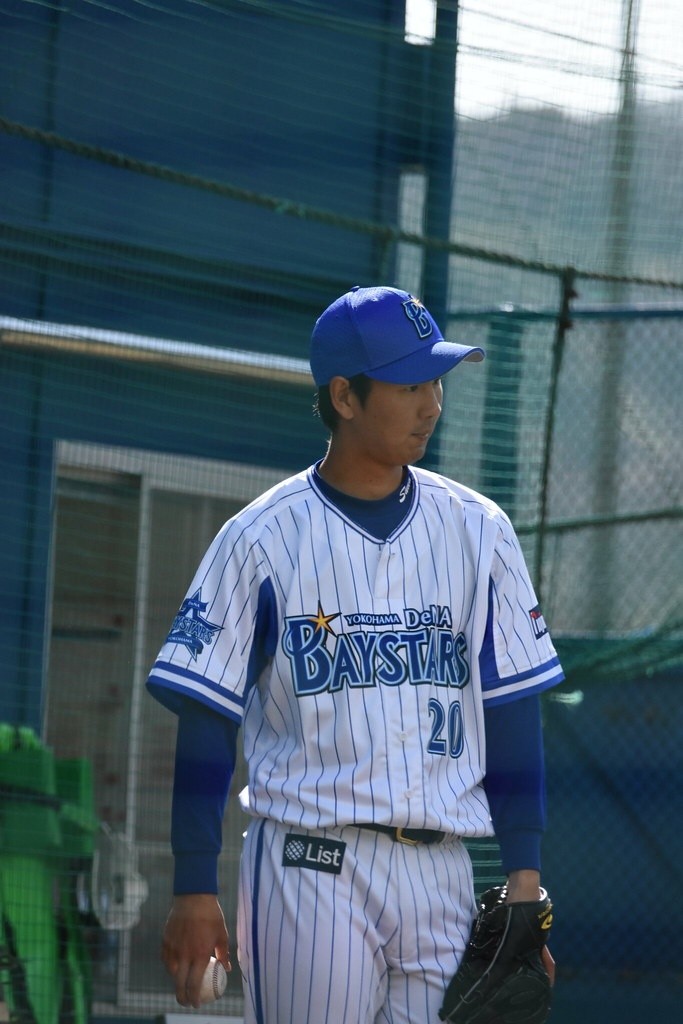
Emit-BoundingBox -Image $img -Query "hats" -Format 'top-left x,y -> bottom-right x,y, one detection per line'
310,286 -> 485,387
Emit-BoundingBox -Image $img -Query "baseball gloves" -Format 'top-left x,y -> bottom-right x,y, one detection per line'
440,883 -> 553,1024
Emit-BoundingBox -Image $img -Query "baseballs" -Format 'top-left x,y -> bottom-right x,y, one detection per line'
193,955 -> 228,1003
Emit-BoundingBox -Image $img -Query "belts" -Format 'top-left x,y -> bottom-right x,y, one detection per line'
346,823 -> 460,846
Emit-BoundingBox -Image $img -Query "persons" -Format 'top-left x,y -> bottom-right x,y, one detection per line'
148,284 -> 563,1024
